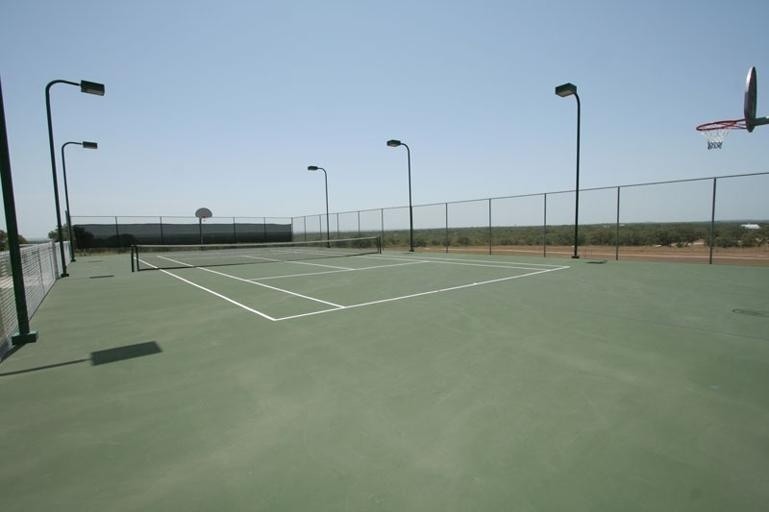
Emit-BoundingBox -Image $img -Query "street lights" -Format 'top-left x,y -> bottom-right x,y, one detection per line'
307,164 -> 331,248
43,75 -> 106,278
59,136 -> 102,265
552,79 -> 580,259
384,139 -> 417,254
